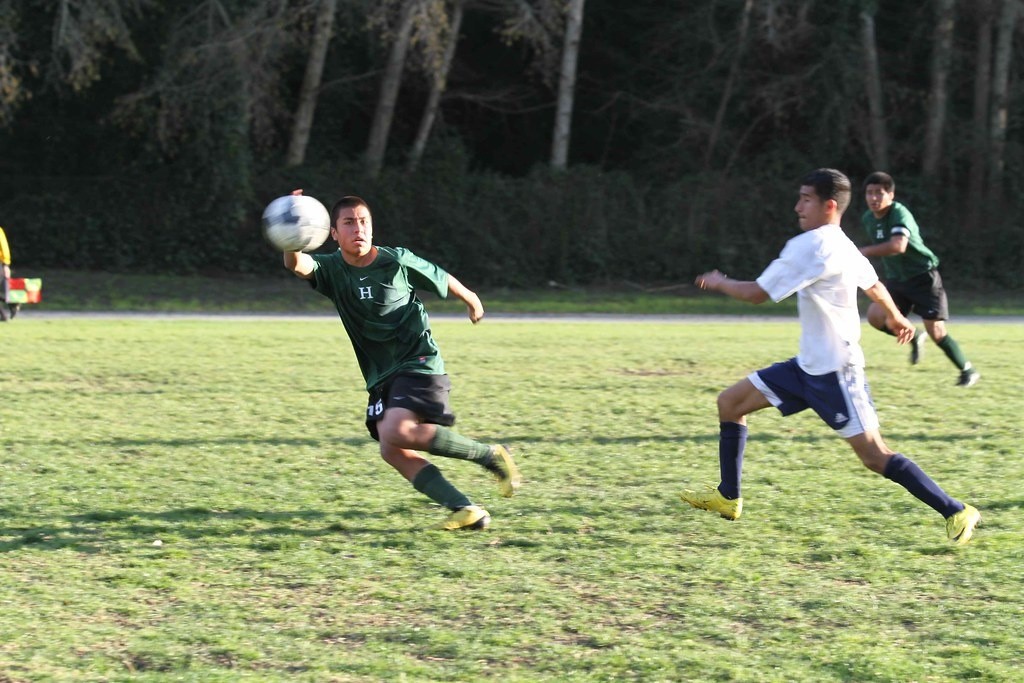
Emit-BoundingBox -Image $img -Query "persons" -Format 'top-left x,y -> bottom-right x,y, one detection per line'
284,189 -> 516,533
858,171 -> 980,387
0,226 -> 20,322
677,166 -> 981,549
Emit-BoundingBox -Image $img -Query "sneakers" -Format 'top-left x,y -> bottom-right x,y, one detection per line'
957,367 -> 981,388
945,504 -> 980,544
679,487 -> 742,522
911,330 -> 927,364
424,504 -> 490,532
485,444 -> 517,497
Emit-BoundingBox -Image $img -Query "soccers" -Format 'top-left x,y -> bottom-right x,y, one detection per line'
262,196 -> 330,252
